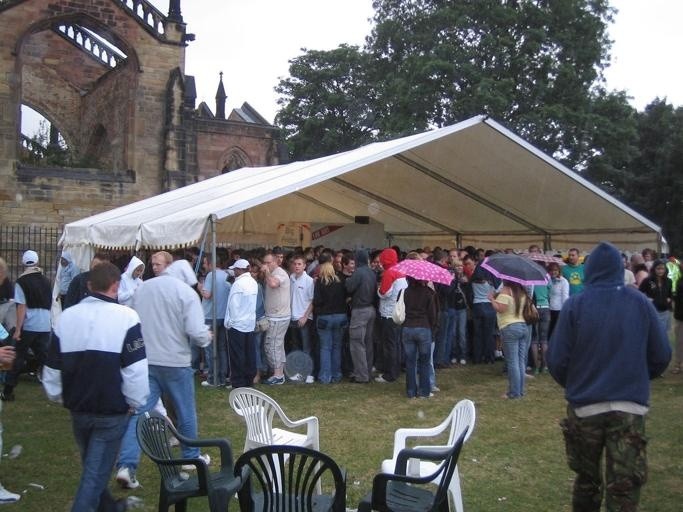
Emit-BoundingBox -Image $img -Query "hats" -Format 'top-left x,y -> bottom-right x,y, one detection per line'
227,259 -> 250,270
21,250 -> 39,266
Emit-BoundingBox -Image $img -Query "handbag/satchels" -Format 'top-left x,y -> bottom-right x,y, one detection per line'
522,295 -> 540,326
390,300 -> 407,325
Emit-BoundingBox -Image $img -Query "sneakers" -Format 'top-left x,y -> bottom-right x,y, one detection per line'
201,379 -> 220,387
115,466 -> 140,491
431,387 -> 440,392
181,453 -> 212,472
420,393 -> 434,399
289,372 -> 304,383
0,393 -> 16,401
114,497 -> 127,512
263,376 -> 286,386
0,484 -> 22,505
374,374 -> 394,383
163,436 -> 180,448
306,375 -> 314,385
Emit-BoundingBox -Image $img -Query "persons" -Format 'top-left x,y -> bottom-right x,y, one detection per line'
616,249 -> 683,377
0,257 -> 22,504
115,258 -> 212,489
544,240 -> 672,512
1,244 -> 589,401
42,264 -> 150,512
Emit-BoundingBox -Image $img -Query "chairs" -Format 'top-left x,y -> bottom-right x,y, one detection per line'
135,411 -> 254,512
228,387 -> 322,499
234,444 -> 348,512
381,399 -> 476,512
357,425 -> 470,512
